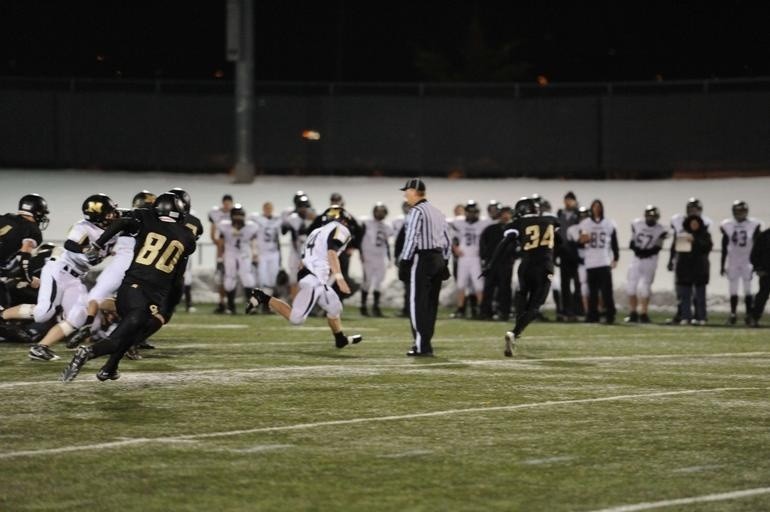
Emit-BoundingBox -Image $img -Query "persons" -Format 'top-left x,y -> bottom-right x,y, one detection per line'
329,194 -> 412,318
208,190 -> 319,317
0,187 -> 204,384
400,180 -> 452,357
450,191 -> 619,356
244,203 -> 363,351
623,199 -> 770,327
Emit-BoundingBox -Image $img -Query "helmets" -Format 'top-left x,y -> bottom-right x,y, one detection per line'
464,194 -> 551,223
230,205 -> 246,224
732,200 -> 748,222
293,191 -> 311,208
82,187 -> 192,231
373,203 -> 387,221
19,193 -> 50,221
685,198 -> 704,215
321,206 -> 352,225
644,206 -> 660,225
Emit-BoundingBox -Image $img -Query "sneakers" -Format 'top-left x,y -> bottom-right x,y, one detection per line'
504,330 -> 517,356
336,335 -> 362,348
245,289 -> 264,313
28,325 -> 155,384
625,311 -> 759,327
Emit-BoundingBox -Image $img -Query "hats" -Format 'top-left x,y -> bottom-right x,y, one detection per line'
399,179 -> 426,191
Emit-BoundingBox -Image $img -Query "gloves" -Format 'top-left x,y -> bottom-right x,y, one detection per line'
398,261 -> 411,281
442,266 -> 450,280
